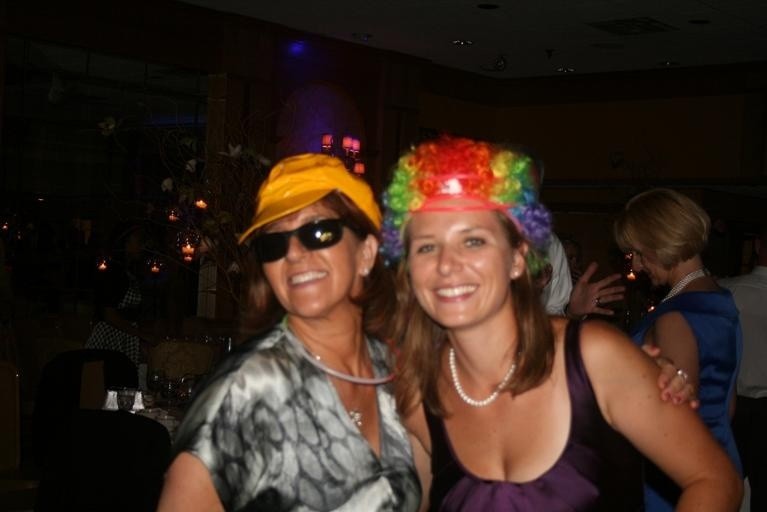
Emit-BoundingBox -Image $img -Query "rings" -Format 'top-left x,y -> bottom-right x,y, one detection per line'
677,369 -> 688,379
596,297 -> 600,304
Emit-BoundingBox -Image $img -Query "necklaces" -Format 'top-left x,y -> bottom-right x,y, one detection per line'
449,348 -> 516,406
662,268 -> 711,303
283,316 -> 395,385
349,408 -> 363,426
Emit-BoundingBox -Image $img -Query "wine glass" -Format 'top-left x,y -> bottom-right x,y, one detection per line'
146,369 -> 177,412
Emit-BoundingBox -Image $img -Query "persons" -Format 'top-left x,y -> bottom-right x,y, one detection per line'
523,158 -> 626,318
84,219 -> 163,368
380,137 -> 745,512
614,188 -> 745,512
157,153 -> 699,512
715,213 -> 767,512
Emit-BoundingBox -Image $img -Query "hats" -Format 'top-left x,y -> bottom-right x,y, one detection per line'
320,232 -> 333,243
238,152 -> 383,247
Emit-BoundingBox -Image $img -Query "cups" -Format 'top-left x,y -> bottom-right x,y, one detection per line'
116,386 -> 136,412
155,415 -> 176,439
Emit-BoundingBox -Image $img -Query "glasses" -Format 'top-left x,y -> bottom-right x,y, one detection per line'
250,214 -> 351,262
